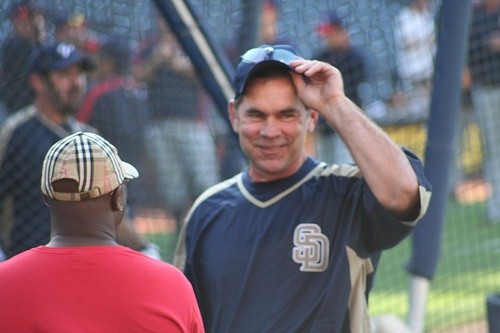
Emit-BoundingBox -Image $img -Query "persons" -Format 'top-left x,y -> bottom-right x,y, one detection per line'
0,0 -> 500,261
174,45 -> 433,333
0,131 -> 205,333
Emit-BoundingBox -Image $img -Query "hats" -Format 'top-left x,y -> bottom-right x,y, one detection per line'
42,131 -> 139,201
231,45 -> 301,101
31,41 -> 96,71
